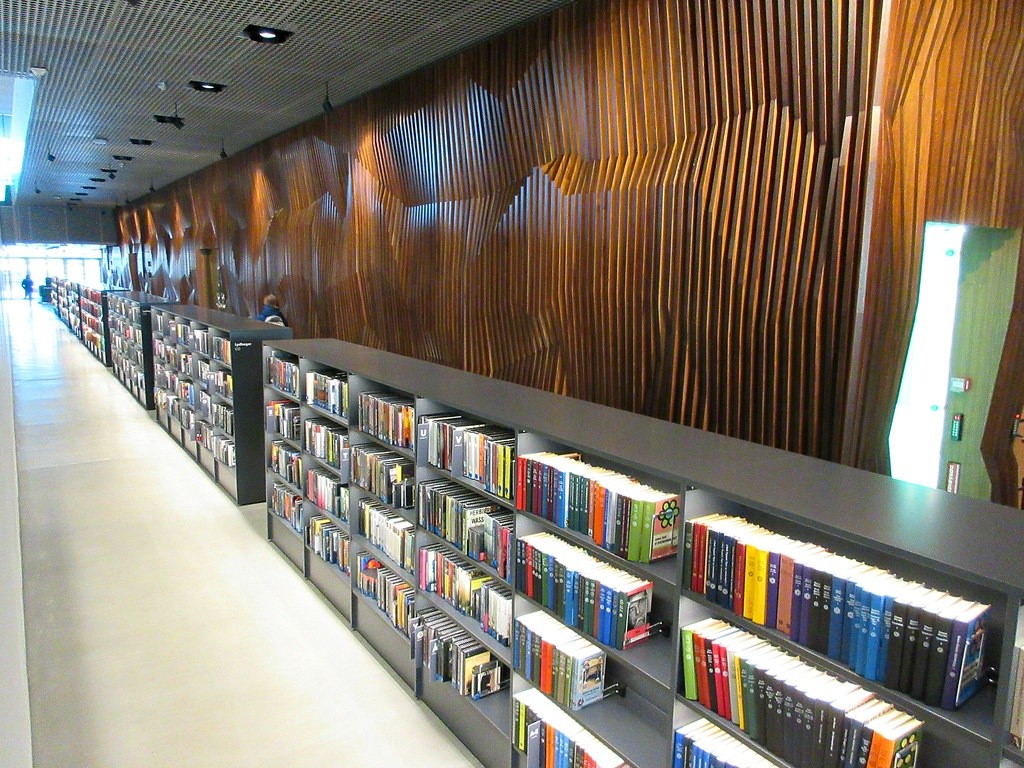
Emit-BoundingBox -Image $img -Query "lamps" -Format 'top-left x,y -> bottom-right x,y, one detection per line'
48,149 -> 56,162
173,102 -> 184,131
35,186 -> 41,195
98,171 -> 156,216
220,138 -> 228,159
322,81 -> 334,112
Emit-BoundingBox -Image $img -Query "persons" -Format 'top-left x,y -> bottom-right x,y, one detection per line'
21,274 -> 34,301
255,294 -> 290,328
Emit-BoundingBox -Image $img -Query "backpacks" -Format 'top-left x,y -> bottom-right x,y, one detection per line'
265,315 -> 285,327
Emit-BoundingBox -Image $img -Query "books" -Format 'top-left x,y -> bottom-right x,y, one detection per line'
152,312 -> 236,469
81,287 -> 105,352
107,300 -> 145,390
266,348 -> 992,768
51,277 -> 81,330
1003,605 -> 1024,768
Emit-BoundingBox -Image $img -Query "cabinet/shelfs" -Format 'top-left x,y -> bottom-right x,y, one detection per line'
45,277 -> 1024,768
